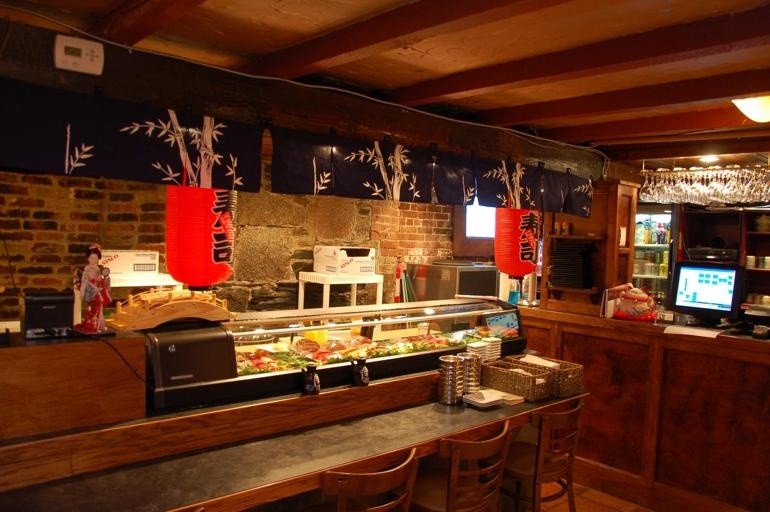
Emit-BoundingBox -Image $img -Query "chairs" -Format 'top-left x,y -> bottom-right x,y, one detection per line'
319,396 -> 583,512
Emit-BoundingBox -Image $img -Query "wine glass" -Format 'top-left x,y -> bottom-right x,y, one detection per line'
638,164 -> 770,206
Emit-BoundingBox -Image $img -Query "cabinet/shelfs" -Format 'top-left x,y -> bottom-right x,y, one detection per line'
671,204 -> 769,326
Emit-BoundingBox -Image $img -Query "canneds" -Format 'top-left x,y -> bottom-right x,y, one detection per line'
652,233 -> 658,244
655,252 -> 661,263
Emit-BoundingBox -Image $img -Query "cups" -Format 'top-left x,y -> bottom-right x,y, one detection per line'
746,253 -> 770,305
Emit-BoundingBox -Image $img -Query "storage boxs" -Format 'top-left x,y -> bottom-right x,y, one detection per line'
97,250 -> 159,274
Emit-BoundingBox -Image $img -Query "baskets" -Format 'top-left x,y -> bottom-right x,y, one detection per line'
481,360 -> 551,403
504,353 -> 584,398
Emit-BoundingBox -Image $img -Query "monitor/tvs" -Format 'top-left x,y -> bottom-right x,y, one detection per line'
550,238 -> 597,289
665,260 -> 743,328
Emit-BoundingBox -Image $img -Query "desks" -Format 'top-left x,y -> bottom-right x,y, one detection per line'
3,400 -> 584,510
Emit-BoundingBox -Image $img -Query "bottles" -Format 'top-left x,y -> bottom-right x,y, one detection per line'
554,213 -> 569,236
648,290 -> 666,313
635,218 -> 670,278
350,357 -> 370,386
301,363 -> 320,395
509,272 -> 537,306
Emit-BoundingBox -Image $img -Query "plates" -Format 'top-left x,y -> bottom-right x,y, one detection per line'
466,337 -> 502,363
462,390 -> 505,409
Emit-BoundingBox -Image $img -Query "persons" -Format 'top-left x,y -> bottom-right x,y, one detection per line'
73,247 -> 111,335
